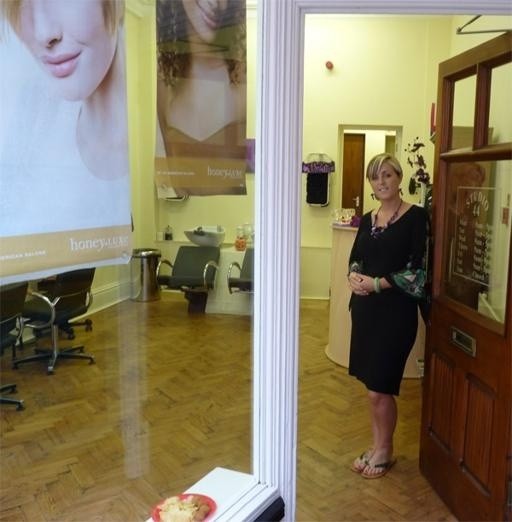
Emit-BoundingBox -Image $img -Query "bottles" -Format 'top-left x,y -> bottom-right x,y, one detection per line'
164,225 -> 173,240
233,225 -> 247,251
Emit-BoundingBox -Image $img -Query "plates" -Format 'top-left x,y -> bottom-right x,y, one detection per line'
151,491 -> 218,522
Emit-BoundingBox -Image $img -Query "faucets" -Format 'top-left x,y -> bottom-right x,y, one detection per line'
216,224 -> 225,232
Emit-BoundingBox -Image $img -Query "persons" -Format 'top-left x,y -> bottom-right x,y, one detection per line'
420,162 -> 487,327
345,151 -> 430,481
157,0 -> 245,198
0,0 -> 125,237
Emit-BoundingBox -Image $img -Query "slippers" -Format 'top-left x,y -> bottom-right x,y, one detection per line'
361,456 -> 397,479
351,447 -> 374,473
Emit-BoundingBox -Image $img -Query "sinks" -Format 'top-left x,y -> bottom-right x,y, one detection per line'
184,225 -> 226,247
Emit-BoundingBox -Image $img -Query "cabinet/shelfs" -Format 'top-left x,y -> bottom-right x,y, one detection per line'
419,29 -> 512,521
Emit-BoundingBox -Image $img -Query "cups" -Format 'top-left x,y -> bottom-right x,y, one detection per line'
330,207 -> 356,225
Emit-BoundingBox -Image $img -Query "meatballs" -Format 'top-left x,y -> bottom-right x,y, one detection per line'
192,496 -> 210,522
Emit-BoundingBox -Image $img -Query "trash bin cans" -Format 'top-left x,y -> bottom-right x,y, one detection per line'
130,248 -> 161,302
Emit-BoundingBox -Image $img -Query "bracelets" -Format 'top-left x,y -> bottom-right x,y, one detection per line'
374,276 -> 380,294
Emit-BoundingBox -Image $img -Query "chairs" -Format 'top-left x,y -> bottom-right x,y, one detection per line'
227,247 -> 255,294
0,267 -> 97,409
155,246 -> 221,291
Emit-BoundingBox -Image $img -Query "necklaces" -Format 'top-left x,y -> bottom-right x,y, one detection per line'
369,199 -> 402,239
